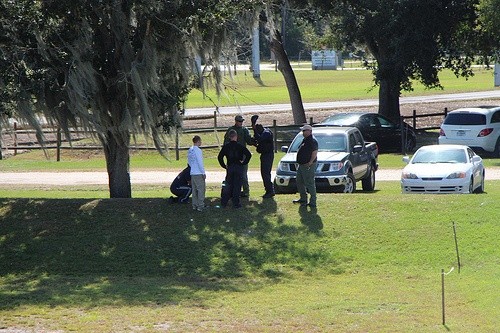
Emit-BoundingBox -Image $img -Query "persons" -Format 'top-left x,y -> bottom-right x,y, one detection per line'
167,163 -> 193,204
186,135 -> 209,212
217,129 -> 252,208
251,124 -> 276,199
292,125 -> 319,206
224,114 -> 256,197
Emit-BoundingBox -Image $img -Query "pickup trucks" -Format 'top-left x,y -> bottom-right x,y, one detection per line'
274,127 -> 378,194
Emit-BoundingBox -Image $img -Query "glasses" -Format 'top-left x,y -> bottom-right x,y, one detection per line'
302,130 -> 307,132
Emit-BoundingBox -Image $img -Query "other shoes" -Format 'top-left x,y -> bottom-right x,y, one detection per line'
233,203 -> 241,208
301,204 -> 316,206
243,191 -> 249,197
193,206 -> 209,212
262,188 -> 275,198
221,201 -> 226,207
293,200 -> 307,203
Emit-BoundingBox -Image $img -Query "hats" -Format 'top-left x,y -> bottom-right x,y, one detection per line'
299,125 -> 312,131
251,115 -> 259,126
235,114 -> 245,122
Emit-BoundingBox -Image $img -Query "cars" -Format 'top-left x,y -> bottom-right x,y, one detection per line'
438,107 -> 500,156
312,113 -> 416,153
401,144 -> 486,195
